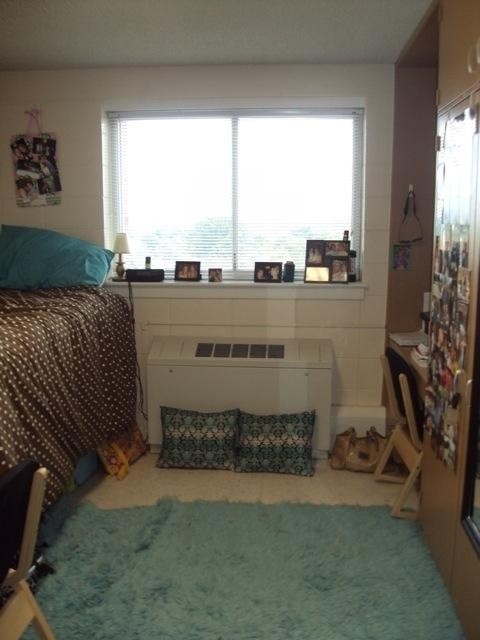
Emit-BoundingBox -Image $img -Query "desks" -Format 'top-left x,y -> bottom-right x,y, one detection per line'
388,335 -> 426,382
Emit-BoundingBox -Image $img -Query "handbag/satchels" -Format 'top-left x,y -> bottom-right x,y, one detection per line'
329,426 -> 395,474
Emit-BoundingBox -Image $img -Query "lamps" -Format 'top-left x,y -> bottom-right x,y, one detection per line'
113,233 -> 130,281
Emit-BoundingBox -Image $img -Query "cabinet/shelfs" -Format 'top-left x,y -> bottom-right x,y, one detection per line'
412,0 -> 480,639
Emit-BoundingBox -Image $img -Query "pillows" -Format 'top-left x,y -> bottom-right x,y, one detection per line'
158,404 -> 240,473
0,225 -> 114,289
240,409 -> 316,476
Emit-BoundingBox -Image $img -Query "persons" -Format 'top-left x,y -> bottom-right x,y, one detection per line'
308,243 -> 348,281
177,264 -> 197,278
269,264 -> 280,279
255,267 -> 266,279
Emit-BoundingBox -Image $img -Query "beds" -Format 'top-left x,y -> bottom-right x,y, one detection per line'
0,286 -> 136,514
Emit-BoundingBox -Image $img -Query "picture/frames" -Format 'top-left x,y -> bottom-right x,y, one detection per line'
254,262 -> 282,283
304,240 -> 350,283
175,261 -> 200,281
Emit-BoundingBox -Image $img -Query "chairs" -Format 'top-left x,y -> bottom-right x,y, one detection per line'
372,347 -> 424,521
0,458 -> 54,640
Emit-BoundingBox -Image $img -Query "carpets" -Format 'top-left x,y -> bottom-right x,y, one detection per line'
18,494 -> 467,639
